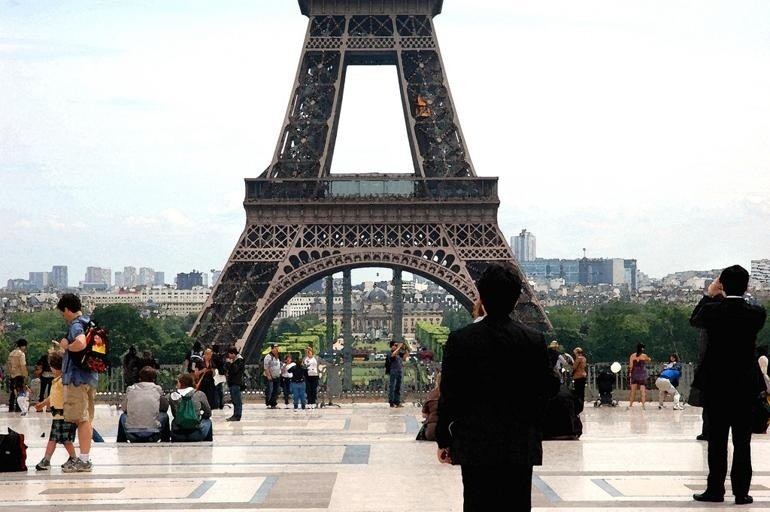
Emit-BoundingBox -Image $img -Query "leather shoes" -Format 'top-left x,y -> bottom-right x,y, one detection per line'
735,496 -> 753,504
693,493 -> 724,502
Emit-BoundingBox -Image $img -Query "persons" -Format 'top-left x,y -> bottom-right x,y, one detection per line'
434,263 -> 560,512
34,351 -> 78,470
123,340 -> 319,421
655,362 -> 684,410
660,354 -> 679,370
166,374 -> 213,442
115,366 -> 171,443
57,293 -> 98,473
8,339 -> 55,413
387,341 -> 409,408
417,341 -> 587,440
695,329 -> 709,441
628,343 -> 651,411
690,264 -> 767,505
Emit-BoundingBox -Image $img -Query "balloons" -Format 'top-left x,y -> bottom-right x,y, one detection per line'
610,362 -> 622,374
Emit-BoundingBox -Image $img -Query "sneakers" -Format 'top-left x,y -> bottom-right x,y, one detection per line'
61,456 -> 77,473
36,458 -> 50,470
65,458 -> 92,472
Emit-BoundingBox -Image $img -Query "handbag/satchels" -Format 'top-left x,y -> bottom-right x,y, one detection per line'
0,428 -> 28,472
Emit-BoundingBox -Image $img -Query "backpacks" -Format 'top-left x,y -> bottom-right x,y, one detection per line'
174,390 -> 198,431
65,318 -> 108,373
